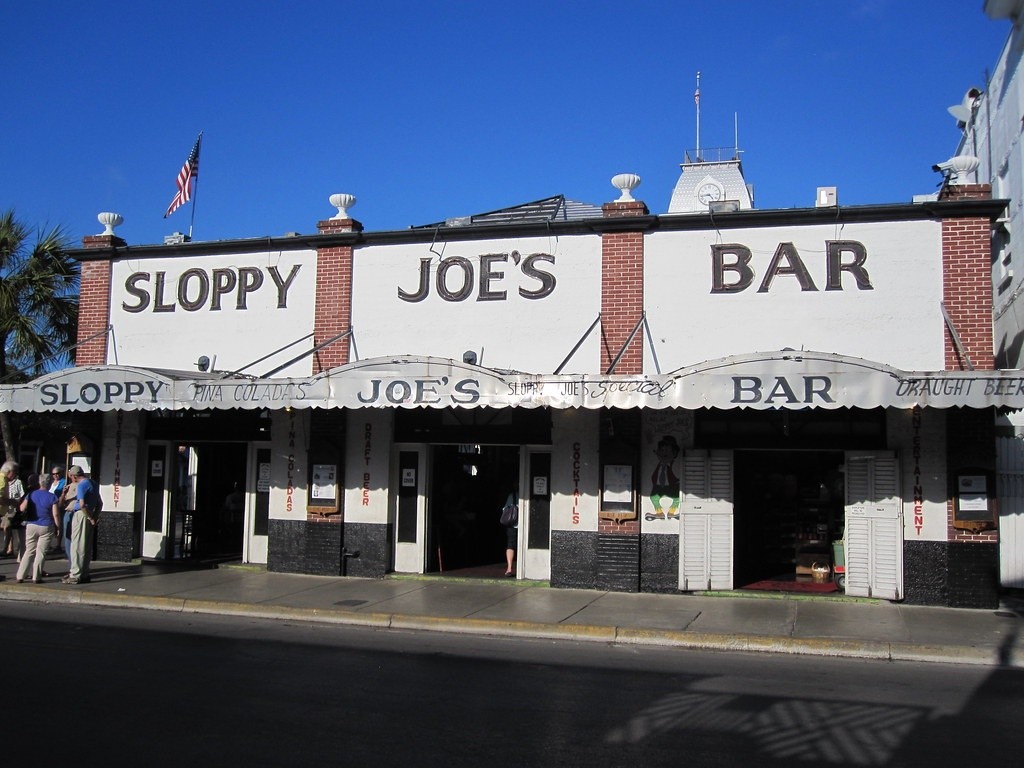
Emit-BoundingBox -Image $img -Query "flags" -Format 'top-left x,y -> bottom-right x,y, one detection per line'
163,135 -> 200,219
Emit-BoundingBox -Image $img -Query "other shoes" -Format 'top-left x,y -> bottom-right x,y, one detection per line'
42,572 -> 50,576
62,578 -> 79,584
16,579 -> 23,583
64,574 -> 69,578
34,580 -> 41,583
79,578 -> 91,583
24,575 -> 32,579
0,575 -> 6,581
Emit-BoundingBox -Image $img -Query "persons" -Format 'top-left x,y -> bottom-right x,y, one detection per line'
505,491 -> 519,576
0,460 -> 104,584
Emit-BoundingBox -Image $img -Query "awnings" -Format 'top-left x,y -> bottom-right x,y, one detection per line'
0,348 -> 1023,412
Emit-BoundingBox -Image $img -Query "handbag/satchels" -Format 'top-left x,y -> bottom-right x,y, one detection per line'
16,491 -> 33,521
500,493 -> 518,526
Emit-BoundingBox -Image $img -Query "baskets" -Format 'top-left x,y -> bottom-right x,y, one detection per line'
811,559 -> 830,584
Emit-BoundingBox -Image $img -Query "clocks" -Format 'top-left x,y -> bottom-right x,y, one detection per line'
698,184 -> 721,206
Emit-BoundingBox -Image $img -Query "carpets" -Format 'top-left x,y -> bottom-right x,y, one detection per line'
742,580 -> 838,593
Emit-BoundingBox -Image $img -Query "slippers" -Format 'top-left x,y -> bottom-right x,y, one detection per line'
505,571 -> 513,577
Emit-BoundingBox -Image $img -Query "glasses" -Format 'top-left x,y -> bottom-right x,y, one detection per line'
52,472 -> 61,476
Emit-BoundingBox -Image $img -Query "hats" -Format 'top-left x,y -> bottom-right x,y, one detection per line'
70,465 -> 84,476
27,474 -> 40,488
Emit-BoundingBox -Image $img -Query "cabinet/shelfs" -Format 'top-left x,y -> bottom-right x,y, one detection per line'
794,502 -> 830,574
